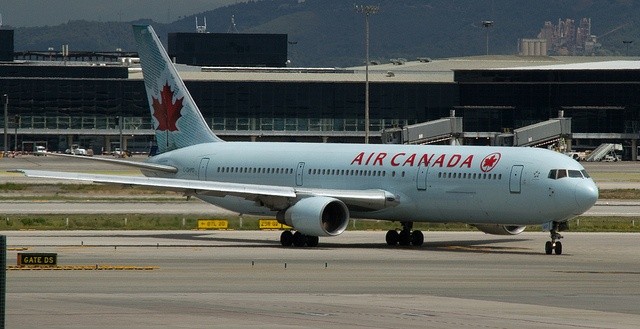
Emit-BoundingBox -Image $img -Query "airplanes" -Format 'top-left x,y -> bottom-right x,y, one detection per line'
6,25 -> 599,254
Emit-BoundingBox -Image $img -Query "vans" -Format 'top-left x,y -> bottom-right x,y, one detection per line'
35,146 -> 46,156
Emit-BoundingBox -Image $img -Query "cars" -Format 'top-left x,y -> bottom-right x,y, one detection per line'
114,148 -> 124,156
